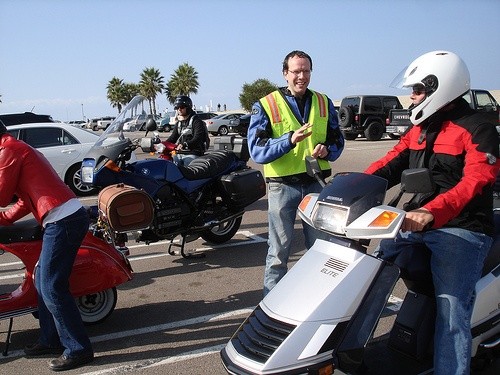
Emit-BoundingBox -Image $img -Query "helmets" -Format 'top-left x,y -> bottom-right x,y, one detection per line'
403,50 -> 469,125
174,95 -> 192,113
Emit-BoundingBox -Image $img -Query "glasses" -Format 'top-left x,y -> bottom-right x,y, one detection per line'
176,105 -> 186,110
287,69 -> 311,75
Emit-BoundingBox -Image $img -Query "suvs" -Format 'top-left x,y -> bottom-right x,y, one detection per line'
337,95 -> 404,141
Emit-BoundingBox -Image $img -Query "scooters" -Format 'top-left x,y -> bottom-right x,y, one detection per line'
0,182 -> 157,357
218,155 -> 500,375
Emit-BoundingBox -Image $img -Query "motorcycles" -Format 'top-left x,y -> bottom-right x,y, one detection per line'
80,94 -> 266,260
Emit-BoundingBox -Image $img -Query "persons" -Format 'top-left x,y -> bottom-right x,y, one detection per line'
0,119 -> 94,371
248,50 -> 344,298
154,95 -> 210,167
365,50 -> 500,375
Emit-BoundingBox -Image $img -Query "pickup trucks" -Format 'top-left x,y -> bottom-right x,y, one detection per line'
154,111 -> 181,133
385,89 -> 500,140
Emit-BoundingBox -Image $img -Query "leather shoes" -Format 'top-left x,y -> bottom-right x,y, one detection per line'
24,342 -> 65,357
49,353 -> 94,371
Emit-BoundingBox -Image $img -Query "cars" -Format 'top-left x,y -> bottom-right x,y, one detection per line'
118,113 -> 162,132
196,112 -> 252,137
0,112 -> 137,197
54,116 -> 116,132
